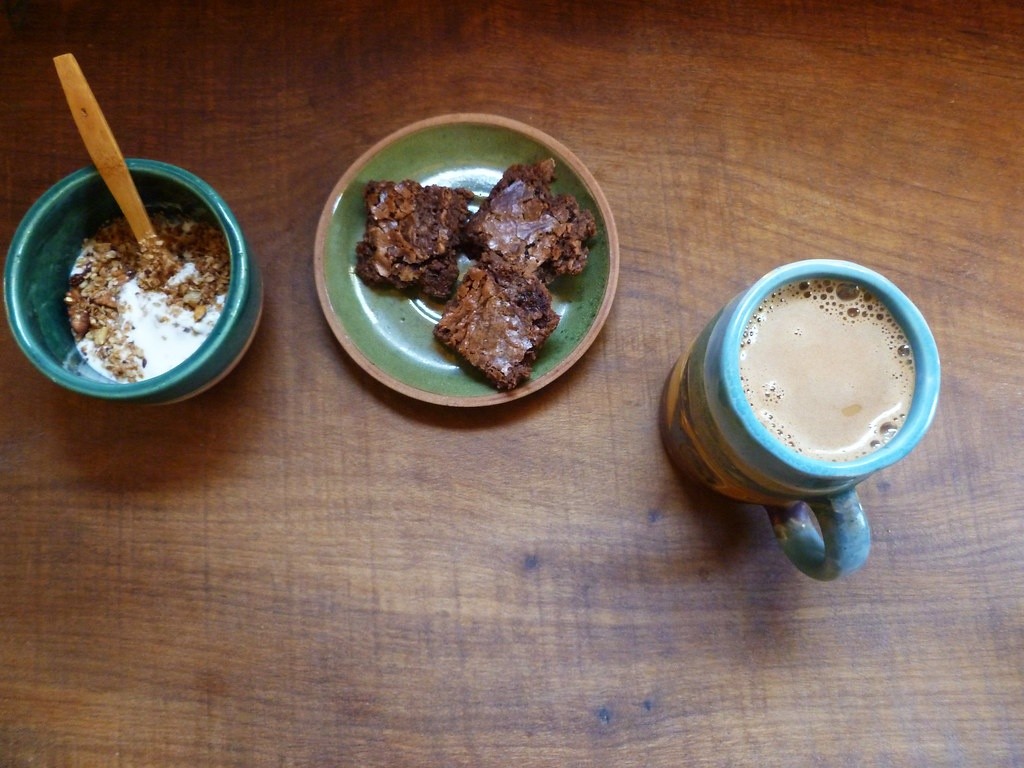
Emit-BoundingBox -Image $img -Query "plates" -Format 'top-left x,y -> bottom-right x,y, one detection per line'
312,112 -> 620,406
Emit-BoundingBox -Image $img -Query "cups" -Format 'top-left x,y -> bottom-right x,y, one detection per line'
5,157 -> 264,405
659,259 -> 941,579
